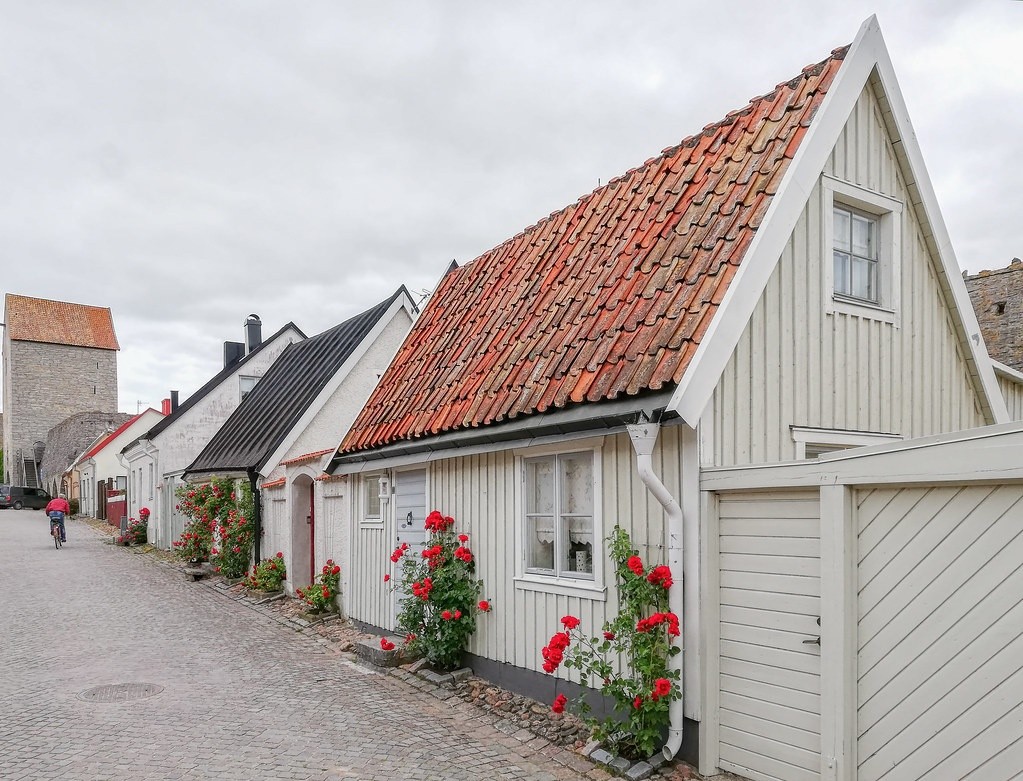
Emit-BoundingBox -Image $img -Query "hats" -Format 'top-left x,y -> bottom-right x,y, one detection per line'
59,494 -> 66,499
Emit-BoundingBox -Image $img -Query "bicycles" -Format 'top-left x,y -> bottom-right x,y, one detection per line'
52,516 -> 65,549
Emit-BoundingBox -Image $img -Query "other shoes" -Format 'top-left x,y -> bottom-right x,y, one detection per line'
51,531 -> 54,534
62,538 -> 65,542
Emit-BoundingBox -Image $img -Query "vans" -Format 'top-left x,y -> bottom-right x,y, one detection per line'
0,484 -> 56,511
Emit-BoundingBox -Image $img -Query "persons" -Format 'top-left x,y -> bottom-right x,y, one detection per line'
45,494 -> 70,541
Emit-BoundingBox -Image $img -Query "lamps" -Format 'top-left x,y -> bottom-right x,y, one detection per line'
406,512 -> 412,525
